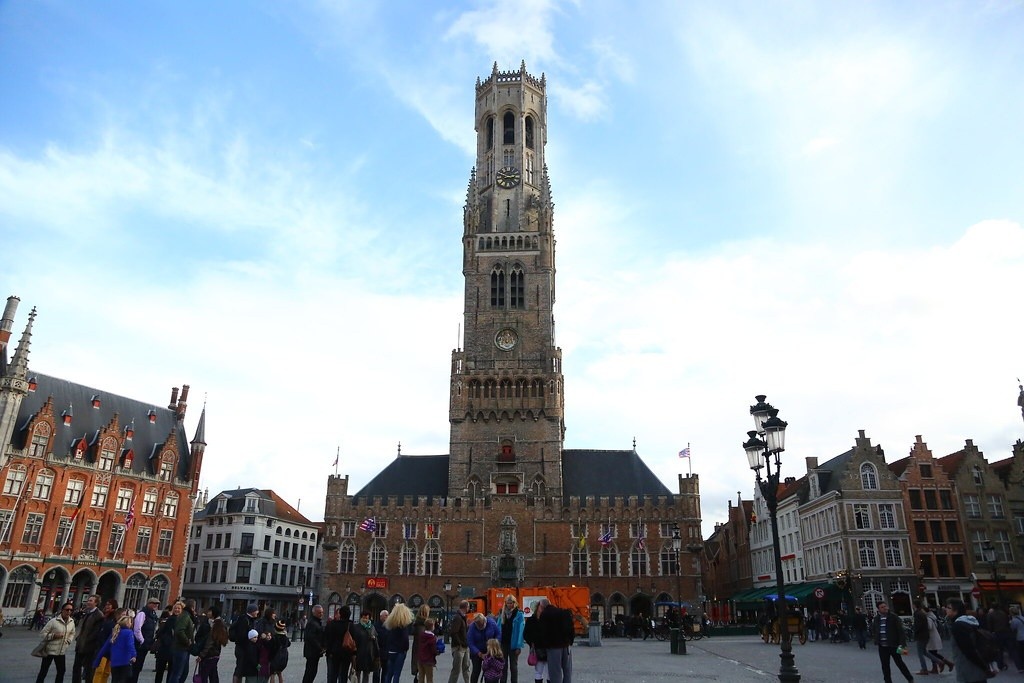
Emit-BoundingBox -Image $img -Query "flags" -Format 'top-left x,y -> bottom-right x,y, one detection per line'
332,449 -> 339,465
637,523 -> 645,549
17,478 -> 27,511
576,529 -> 586,549
359,517 -> 375,533
679,448 -> 689,458
69,488 -> 87,521
125,496 -> 137,531
597,532 -> 612,547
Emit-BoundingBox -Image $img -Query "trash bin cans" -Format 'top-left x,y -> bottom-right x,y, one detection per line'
588,621 -> 602,646
670,628 -> 679,654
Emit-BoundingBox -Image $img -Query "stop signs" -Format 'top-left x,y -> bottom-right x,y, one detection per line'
972,588 -> 981,599
919,584 -> 925,592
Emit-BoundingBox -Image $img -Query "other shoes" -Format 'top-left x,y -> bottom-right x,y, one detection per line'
916,669 -> 928,675
938,660 -> 945,673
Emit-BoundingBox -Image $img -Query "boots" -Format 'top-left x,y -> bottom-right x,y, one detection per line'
928,662 -> 940,674
943,658 -> 954,672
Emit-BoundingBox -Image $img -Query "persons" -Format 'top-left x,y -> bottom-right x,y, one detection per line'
701,613 -> 713,638
28,598 -> 75,683
808,605 -> 867,650
870,601 -> 914,683
910,600 -> 1024,683
72,594 -> 574,683
0,608 -> 4,638
604,617 -> 615,638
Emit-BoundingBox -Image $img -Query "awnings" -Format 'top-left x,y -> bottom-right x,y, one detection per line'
732,583 -> 823,604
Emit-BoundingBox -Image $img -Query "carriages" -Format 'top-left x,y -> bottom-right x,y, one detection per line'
756,594 -> 809,645
615,602 -> 706,642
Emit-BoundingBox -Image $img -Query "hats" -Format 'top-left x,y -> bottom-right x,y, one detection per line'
248,629 -> 258,640
246,603 -> 259,614
276,619 -> 287,630
147,597 -> 162,604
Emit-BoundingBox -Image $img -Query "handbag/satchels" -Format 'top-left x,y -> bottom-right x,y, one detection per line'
342,621 -> 358,653
93,656 -> 112,683
31,630 -> 51,659
191,659 -> 202,683
527,647 -> 538,667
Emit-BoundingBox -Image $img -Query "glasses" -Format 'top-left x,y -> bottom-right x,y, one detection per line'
62,608 -> 74,612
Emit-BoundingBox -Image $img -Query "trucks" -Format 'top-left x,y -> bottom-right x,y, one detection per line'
466,587 -> 590,635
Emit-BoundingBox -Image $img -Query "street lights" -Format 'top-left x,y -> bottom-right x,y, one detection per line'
442,579 -> 450,644
981,540 -> 1005,612
291,584 -> 303,642
741,394 -> 802,683
672,522 -> 687,655
